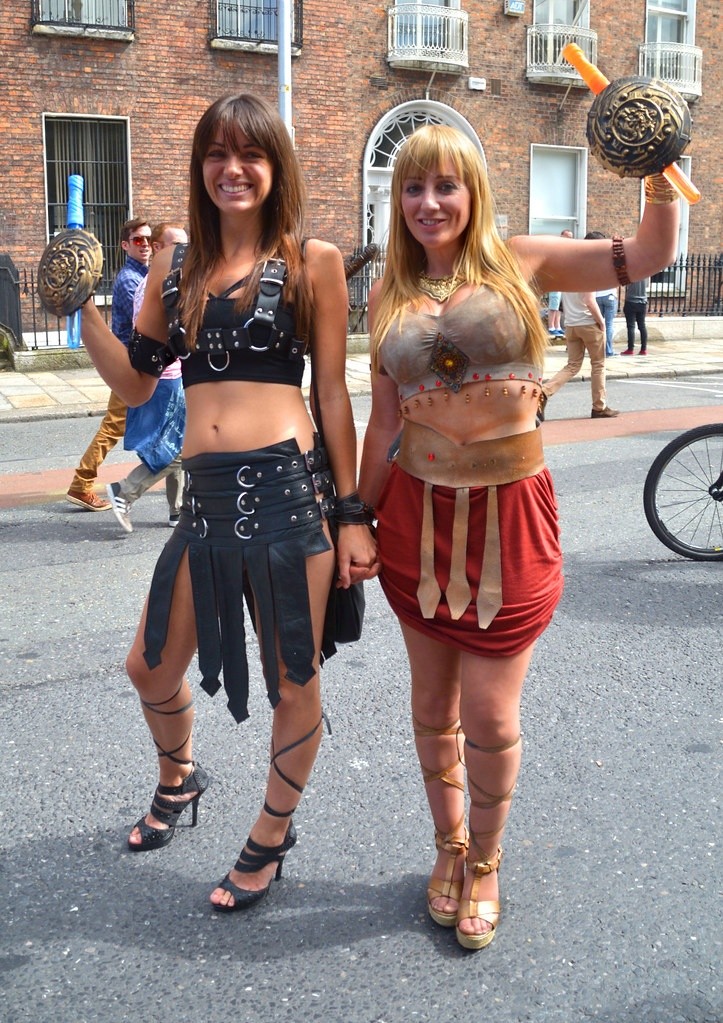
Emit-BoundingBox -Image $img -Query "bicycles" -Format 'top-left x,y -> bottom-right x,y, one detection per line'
642,424 -> 722,561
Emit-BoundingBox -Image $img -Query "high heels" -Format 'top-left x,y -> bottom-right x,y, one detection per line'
127,761 -> 209,851
213,817 -> 297,913
428,825 -> 470,927
455,844 -> 502,948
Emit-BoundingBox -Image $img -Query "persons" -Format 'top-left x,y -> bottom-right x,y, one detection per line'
65,216 -> 189,533
535,231 -> 622,425
594,276 -> 647,356
49,95 -> 380,912
546,230 -> 573,337
338,123 -> 679,950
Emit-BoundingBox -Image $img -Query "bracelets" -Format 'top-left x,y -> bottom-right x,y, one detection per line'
335,491 -> 377,525
639,173 -> 679,205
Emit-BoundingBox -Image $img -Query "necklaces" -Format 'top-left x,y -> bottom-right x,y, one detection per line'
412,267 -> 468,302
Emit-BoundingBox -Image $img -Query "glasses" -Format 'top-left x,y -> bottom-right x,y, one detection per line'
127,236 -> 151,246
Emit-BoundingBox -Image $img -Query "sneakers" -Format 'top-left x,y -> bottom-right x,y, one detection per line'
65,489 -> 112,512
105,482 -> 134,534
168,515 -> 180,526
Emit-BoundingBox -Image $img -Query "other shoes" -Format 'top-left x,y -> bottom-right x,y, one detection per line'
536,391 -> 548,422
620,349 -> 634,356
548,330 -> 561,335
639,350 -> 647,355
557,330 -> 565,334
591,406 -> 620,418
